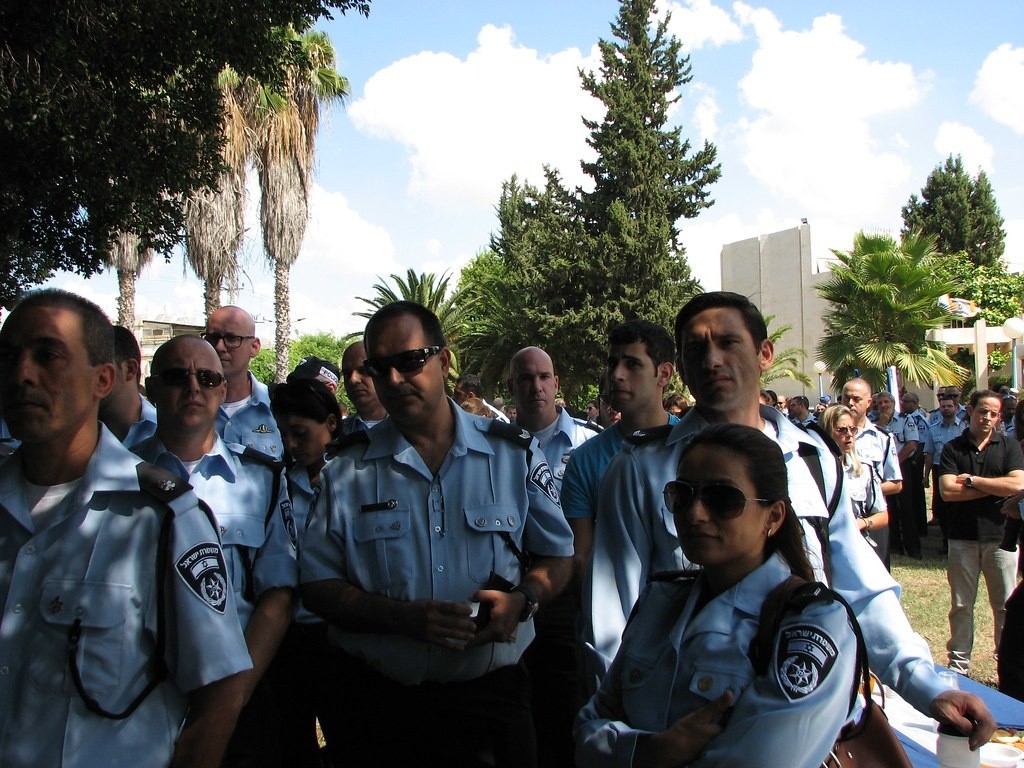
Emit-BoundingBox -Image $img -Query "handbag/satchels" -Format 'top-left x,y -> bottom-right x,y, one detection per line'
751,583 -> 913,768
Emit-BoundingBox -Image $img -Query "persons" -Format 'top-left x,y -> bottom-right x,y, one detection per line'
560,318 -> 679,607
573,423 -> 856,768
97,308 -> 389,768
451,347 -> 621,492
759,378 -> 1024,702
663,393 -> 693,419
298,301 -> 575,768
588,292 -> 998,751
0,290 -> 253,768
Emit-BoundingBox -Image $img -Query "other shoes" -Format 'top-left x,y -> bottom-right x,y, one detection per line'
927,518 -> 941,525
938,546 -> 948,553
907,551 -> 923,559
890,545 -> 904,556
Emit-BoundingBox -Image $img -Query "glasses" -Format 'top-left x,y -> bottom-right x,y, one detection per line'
364,345 -> 443,377
428,475 -> 448,538
149,367 -> 225,390
945,394 -> 960,400
201,333 -> 255,352
597,393 -> 610,406
900,399 -> 915,406
585,407 -> 596,412
832,426 -> 858,436
273,383 -> 327,406
793,395 -> 805,406
662,482 -> 772,520
937,393 -> 946,398
1002,395 -> 1017,401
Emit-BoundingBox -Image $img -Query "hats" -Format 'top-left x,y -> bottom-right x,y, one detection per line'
821,395 -> 831,405
287,356 -> 340,390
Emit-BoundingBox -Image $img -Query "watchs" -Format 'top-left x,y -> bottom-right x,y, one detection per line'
511,585 -> 539,623
965,475 -> 974,487
862,517 -> 873,530
